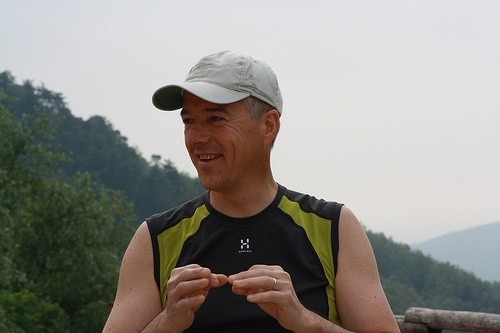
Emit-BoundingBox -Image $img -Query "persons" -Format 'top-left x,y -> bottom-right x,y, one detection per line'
102,51 -> 403,332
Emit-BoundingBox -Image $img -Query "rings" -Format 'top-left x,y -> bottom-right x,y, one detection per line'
272,276 -> 278,291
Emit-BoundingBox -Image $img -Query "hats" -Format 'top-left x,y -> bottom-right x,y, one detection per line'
152,50 -> 284,115
